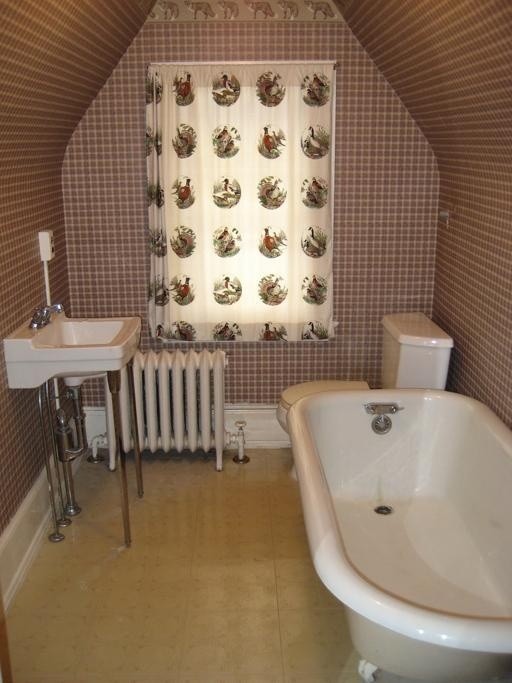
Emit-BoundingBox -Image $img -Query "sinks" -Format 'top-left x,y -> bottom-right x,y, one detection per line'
35,316 -> 143,389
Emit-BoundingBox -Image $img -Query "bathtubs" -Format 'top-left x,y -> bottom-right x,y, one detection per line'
286,388 -> 512,683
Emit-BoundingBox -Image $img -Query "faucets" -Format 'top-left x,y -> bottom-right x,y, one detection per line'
31,303 -> 63,330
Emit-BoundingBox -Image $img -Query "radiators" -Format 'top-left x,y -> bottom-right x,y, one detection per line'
87,348 -> 250,472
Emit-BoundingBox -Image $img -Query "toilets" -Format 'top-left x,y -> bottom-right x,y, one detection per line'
277,313 -> 454,480
